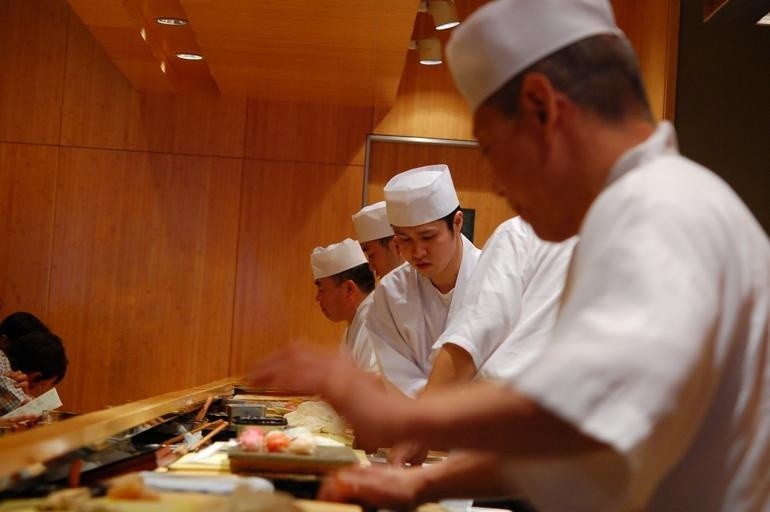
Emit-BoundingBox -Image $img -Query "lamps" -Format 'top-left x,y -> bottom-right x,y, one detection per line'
427,1 -> 460,30
417,37 -> 442,64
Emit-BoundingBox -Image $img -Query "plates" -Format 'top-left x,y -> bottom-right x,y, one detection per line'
140,471 -> 275,493
226,447 -> 360,473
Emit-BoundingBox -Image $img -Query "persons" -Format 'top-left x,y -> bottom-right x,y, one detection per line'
385,212 -> 579,470
351,201 -> 408,279
364,163 -> 484,400
310,237 -> 382,376
0,311 -> 68,424
244,0 -> 770,512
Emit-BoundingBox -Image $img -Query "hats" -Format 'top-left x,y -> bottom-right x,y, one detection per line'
310,238 -> 369,280
383,164 -> 459,228
351,200 -> 395,244
445,0 -> 622,109
